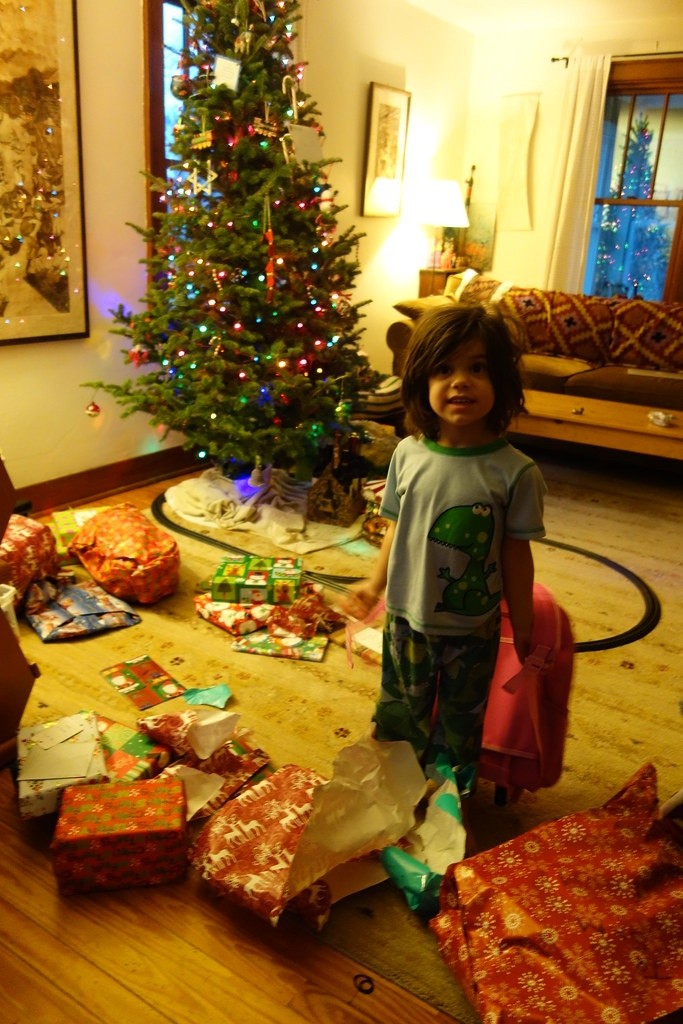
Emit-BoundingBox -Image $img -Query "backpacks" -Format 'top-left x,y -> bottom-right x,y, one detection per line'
429,580 -> 573,806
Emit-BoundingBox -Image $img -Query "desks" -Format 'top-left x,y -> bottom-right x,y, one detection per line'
419,267 -> 470,297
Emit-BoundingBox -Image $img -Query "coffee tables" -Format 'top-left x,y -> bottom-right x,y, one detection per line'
498,388 -> 683,460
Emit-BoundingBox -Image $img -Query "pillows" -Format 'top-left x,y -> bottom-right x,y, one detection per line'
495,285 -> 622,361
603,297 -> 683,371
391,296 -> 457,320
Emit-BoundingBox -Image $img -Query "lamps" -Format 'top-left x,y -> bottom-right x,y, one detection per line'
411,178 -> 471,295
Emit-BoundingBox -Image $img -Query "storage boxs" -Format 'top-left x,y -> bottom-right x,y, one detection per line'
73,709 -> 174,784
17,709 -> 103,820
52,781 -> 190,894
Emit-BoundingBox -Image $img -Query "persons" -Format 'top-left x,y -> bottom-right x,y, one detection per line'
344,302 -> 548,813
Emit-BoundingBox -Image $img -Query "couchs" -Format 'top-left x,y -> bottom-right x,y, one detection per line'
387,282 -> 683,406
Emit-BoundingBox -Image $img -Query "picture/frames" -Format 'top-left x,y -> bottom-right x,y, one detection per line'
1,0 -> 92,343
362,80 -> 411,218
